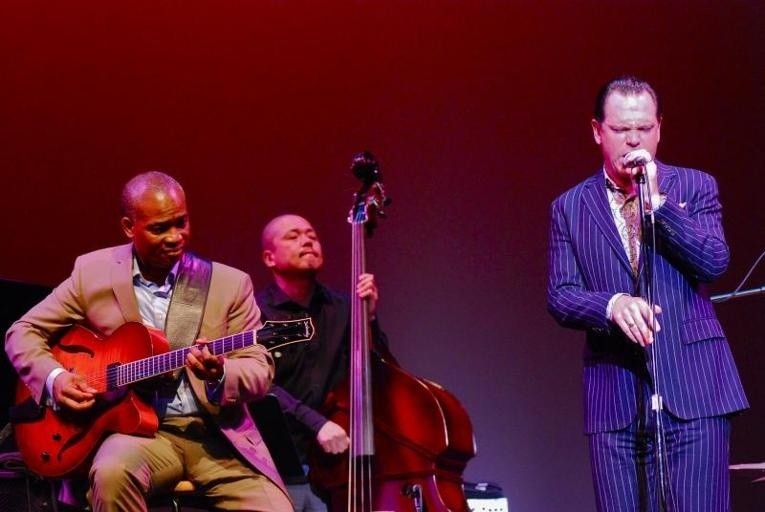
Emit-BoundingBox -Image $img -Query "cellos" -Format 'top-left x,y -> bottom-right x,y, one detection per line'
306,150 -> 478,512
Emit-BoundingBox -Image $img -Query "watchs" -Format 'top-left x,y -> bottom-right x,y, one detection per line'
208,376 -> 222,385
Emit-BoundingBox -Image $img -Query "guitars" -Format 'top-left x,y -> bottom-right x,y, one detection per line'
14,311 -> 317,478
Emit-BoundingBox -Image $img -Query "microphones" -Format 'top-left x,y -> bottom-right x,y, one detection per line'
631,156 -> 645,167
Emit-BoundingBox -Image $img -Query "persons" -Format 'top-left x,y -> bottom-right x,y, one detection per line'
254,214 -> 390,512
4,171 -> 295,512
546,77 -> 751,512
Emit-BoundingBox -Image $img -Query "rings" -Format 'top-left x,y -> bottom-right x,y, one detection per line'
645,159 -> 653,163
627,322 -> 636,328
